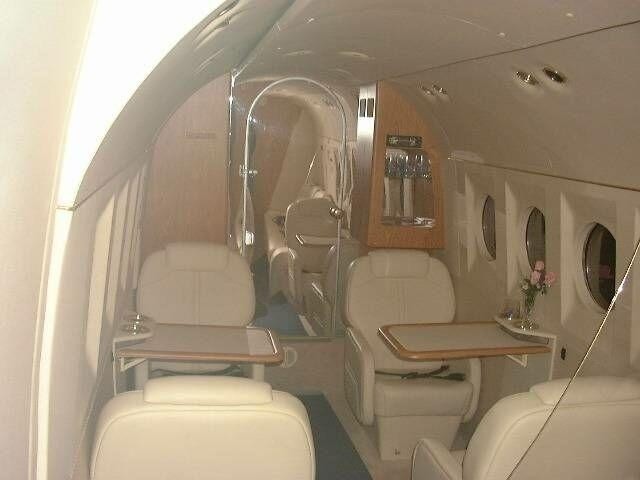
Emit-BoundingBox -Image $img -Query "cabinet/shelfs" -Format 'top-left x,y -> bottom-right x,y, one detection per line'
349,82 -> 445,250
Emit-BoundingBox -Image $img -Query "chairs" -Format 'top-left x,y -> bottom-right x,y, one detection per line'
341,249 -> 482,461
285,197 -> 338,312
134,240 -> 265,390
89,375 -> 316,480
411,375 -> 640,480
302,239 -> 361,337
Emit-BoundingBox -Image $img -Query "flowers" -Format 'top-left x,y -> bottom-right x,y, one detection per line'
521,261 -> 557,320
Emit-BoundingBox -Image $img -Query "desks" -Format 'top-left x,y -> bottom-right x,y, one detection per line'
295,233 -> 338,247
378,321 -> 556,380
112,323 -> 285,396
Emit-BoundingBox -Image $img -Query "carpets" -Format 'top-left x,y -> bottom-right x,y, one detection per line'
292,393 -> 373,480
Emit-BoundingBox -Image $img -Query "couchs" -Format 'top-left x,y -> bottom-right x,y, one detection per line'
263,184 -> 331,304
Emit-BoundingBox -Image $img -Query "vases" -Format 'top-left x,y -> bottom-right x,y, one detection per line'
515,295 -> 540,330
